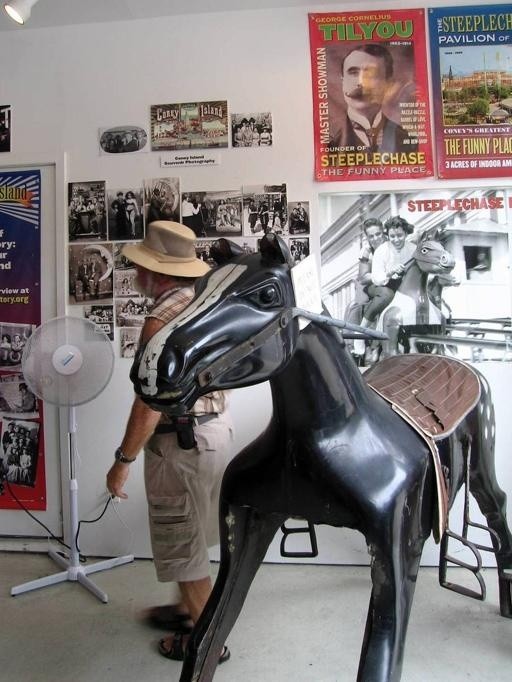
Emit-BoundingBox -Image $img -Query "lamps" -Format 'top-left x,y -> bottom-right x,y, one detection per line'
5,1 -> 39,25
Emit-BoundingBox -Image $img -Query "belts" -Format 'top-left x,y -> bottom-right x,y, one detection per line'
155,411 -> 218,434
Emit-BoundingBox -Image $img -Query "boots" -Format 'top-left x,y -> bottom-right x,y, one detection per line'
353,317 -> 375,354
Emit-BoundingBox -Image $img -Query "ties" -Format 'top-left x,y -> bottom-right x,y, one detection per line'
353,114 -> 386,153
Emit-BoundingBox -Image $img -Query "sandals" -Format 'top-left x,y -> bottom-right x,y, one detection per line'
158,630 -> 229,662
140,601 -> 194,632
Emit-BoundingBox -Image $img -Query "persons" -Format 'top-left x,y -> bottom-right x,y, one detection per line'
98,217 -> 237,670
467,247 -> 491,282
322,41 -> 421,168
341,217 -> 390,362
67,186 -> 310,359
0,323 -> 43,489
351,215 -> 419,356
100,118 -> 272,151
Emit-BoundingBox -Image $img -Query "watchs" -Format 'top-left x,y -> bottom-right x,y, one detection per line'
113,445 -> 137,464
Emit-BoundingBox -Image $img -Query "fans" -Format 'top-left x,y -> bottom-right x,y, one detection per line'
9,313 -> 136,603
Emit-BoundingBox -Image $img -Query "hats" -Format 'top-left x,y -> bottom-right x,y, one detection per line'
122,219 -> 213,279
